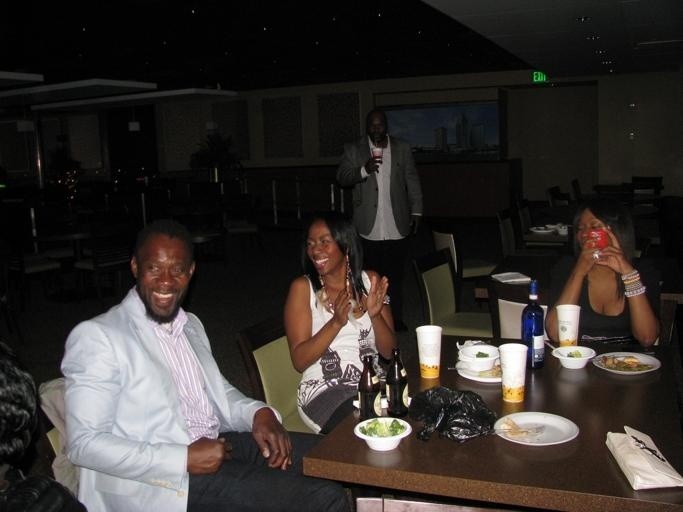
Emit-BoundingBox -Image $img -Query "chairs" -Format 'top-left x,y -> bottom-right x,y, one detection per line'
1,237 -> 62,313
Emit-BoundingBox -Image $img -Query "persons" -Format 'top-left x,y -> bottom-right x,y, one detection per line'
282,209 -> 398,436
335,108 -> 423,334
0,341 -> 88,512
60,220 -> 355,512
543,199 -> 661,345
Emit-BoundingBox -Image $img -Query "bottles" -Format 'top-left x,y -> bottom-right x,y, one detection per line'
386,347 -> 409,414
358,356 -> 380,419
520,278 -> 543,366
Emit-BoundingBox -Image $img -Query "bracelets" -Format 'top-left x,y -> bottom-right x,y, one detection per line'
621,269 -> 646,297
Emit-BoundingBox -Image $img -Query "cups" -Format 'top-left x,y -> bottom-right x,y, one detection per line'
555,304 -> 581,347
372,147 -> 383,166
413,326 -> 442,378
498,343 -> 528,401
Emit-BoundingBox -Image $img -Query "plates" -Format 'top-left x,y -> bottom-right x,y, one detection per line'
493,410 -> 577,445
528,225 -> 555,235
590,351 -> 661,374
454,360 -> 502,384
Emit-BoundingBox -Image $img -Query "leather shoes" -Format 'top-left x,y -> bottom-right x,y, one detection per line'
394,319 -> 408,333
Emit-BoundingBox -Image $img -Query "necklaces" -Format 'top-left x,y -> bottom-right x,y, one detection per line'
319,272 -> 353,316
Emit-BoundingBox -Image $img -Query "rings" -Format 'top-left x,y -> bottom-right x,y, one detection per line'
592,250 -> 603,260
383,294 -> 392,305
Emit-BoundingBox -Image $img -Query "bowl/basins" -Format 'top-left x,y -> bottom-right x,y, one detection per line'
551,346 -> 592,370
461,346 -> 498,374
351,416 -> 413,452
